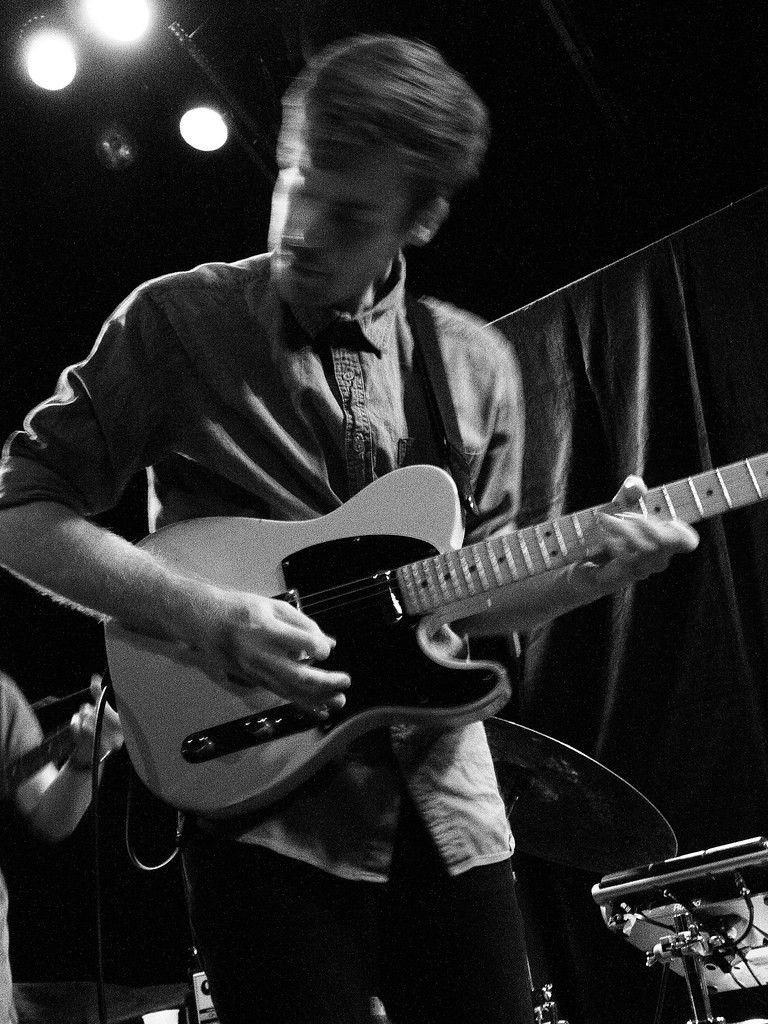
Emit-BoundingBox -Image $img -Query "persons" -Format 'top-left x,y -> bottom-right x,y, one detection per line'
0,37 -> 700,1024
1,665 -> 127,1024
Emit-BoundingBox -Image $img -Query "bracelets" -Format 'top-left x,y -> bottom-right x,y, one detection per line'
67,745 -> 110,774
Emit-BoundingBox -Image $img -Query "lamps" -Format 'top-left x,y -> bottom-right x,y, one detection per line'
19,0 -> 240,172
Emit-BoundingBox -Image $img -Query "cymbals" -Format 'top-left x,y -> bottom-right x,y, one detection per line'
482,714 -> 679,877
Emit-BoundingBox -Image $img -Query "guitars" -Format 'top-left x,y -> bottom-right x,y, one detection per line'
103,451 -> 768,820
